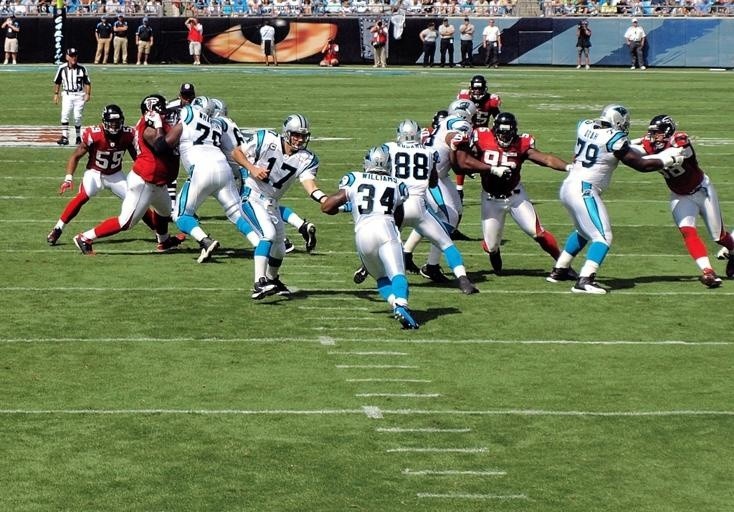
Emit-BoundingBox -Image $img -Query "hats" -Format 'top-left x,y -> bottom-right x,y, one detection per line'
631,18 -> 639,23
66,47 -> 79,57
118,13 -> 125,17
100,15 -> 108,20
143,17 -> 149,22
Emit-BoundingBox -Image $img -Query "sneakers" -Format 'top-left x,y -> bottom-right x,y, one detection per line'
354,248 -> 503,331
372,63 -> 387,68
71,232 -> 96,257
4,59 -> 17,65
421,63 -> 499,69
699,267 -> 722,288
717,232 -> 734,279
46,226 -> 63,244
575,64 -> 591,71
546,266 -> 578,283
571,273 -> 607,295
57,135 -> 83,144
93,60 -> 149,66
630,65 -> 648,71
193,61 -> 201,66
156,222 -> 319,300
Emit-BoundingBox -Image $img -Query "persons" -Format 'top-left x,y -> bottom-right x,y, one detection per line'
0,1 -> 733,70
52,47 -> 91,146
322,75 -> 575,332
46,82 -> 328,299
625,114 -> 734,291
547,104 -> 686,298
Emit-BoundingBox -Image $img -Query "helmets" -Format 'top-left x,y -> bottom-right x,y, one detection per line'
101,83 -> 228,134
363,146 -> 393,175
396,76 -> 518,147
647,113 -> 675,150
283,112 -> 311,152
599,104 -> 630,136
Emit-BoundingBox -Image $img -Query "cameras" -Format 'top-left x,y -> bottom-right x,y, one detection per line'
378,22 -> 382,26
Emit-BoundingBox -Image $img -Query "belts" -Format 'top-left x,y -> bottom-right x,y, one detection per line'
489,189 -> 521,200
65,88 -> 83,93
